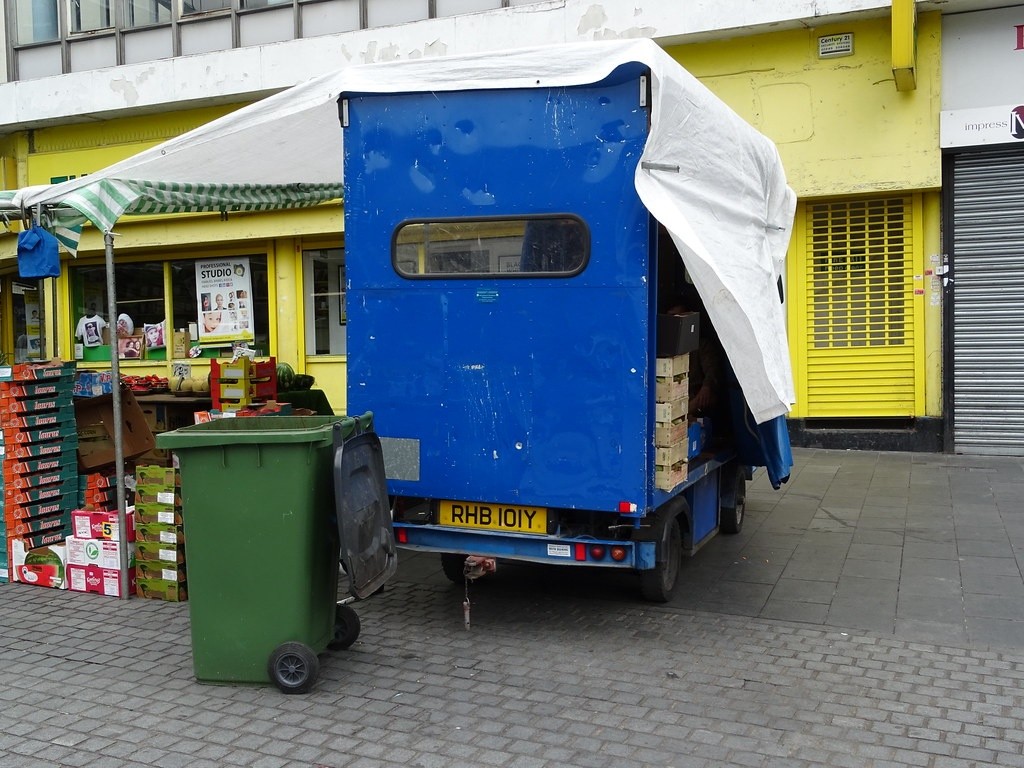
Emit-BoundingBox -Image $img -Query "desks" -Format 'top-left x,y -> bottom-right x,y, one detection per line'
134,390 -> 335,416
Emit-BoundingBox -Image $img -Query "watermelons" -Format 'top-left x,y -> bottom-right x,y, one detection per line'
15,550 -> 66,588
275,362 -> 294,393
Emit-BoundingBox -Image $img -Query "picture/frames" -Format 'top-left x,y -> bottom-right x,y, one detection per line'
118,334 -> 145,359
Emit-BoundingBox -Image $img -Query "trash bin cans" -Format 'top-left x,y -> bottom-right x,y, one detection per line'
156,416 -> 399,694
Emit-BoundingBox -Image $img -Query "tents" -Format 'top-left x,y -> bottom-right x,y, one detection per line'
0,175 -> 344,600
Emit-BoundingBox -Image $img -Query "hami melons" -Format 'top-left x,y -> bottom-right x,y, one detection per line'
168,376 -> 209,391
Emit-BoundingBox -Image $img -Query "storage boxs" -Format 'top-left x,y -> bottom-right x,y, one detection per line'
0,362 -> 188,603
210,355 -> 277,413
652,309 -> 714,493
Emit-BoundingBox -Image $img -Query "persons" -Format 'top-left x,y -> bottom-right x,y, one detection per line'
199,293 -> 210,311
120,341 -> 141,358
213,294 -> 226,310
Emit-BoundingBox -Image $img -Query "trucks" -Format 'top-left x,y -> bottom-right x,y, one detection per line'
343,41 -> 790,607
